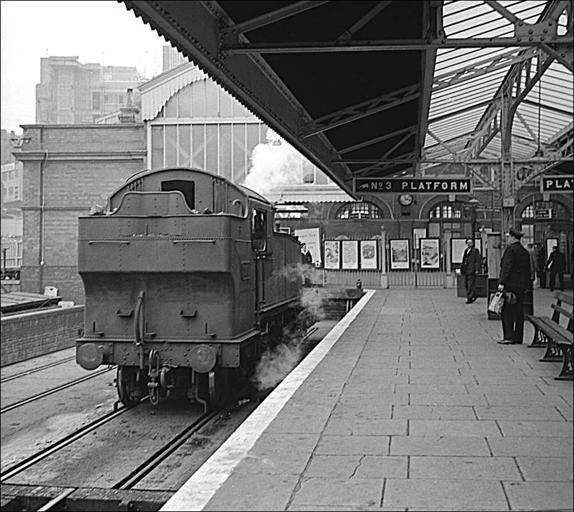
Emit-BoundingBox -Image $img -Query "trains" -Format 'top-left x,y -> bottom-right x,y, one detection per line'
73,166 -> 312,412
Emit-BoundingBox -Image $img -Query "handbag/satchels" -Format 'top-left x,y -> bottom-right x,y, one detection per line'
487,289 -> 522,321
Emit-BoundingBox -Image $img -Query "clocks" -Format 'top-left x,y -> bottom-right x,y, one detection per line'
399,195 -> 413,206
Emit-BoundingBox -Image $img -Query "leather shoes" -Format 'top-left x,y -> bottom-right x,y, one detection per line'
497,338 -> 523,344
466,296 -> 477,304
538,285 -> 554,292
561,288 -> 564,291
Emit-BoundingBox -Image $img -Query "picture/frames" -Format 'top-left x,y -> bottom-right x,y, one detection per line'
390,238 -> 410,271
450,237 -> 482,264
324,240 -> 341,270
359,239 -> 379,272
341,240 -> 359,271
420,238 -> 440,270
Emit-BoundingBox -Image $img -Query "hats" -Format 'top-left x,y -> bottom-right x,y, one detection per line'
509,227 -> 525,240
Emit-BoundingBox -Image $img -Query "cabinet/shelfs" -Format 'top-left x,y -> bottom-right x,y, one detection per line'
457,275 -> 488,297
486,278 -> 533,321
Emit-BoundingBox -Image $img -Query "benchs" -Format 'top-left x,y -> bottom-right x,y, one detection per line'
528,292 -> 574,380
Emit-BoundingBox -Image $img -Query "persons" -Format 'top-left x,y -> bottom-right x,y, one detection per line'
527,243 -> 545,288
301,243 -> 311,289
546,246 -> 564,292
461,238 -> 482,304
497,227 -> 531,345
